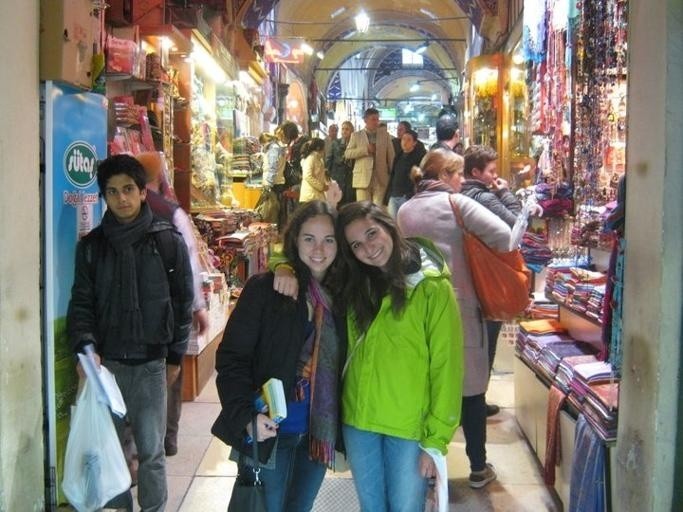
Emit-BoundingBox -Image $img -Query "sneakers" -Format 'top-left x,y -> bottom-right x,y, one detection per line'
469,463 -> 498,491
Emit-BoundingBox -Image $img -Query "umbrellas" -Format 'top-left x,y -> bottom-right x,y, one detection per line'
508,198 -> 543,251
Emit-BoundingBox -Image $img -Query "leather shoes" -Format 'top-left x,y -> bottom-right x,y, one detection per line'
485,403 -> 500,418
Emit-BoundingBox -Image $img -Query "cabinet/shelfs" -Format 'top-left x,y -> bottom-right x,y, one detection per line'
507,203 -> 624,511
104,70 -> 162,141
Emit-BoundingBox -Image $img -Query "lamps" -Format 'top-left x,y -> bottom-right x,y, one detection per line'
414,39 -> 429,56
406,76 -> 459,95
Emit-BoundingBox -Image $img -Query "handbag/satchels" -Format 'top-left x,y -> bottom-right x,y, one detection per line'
211,404 -> 281,465
322,179 -> 344,204
421,445 -> 449,512
253,186 -> 282,223
226,476 -> 266,512
460,232 -> 533,325
